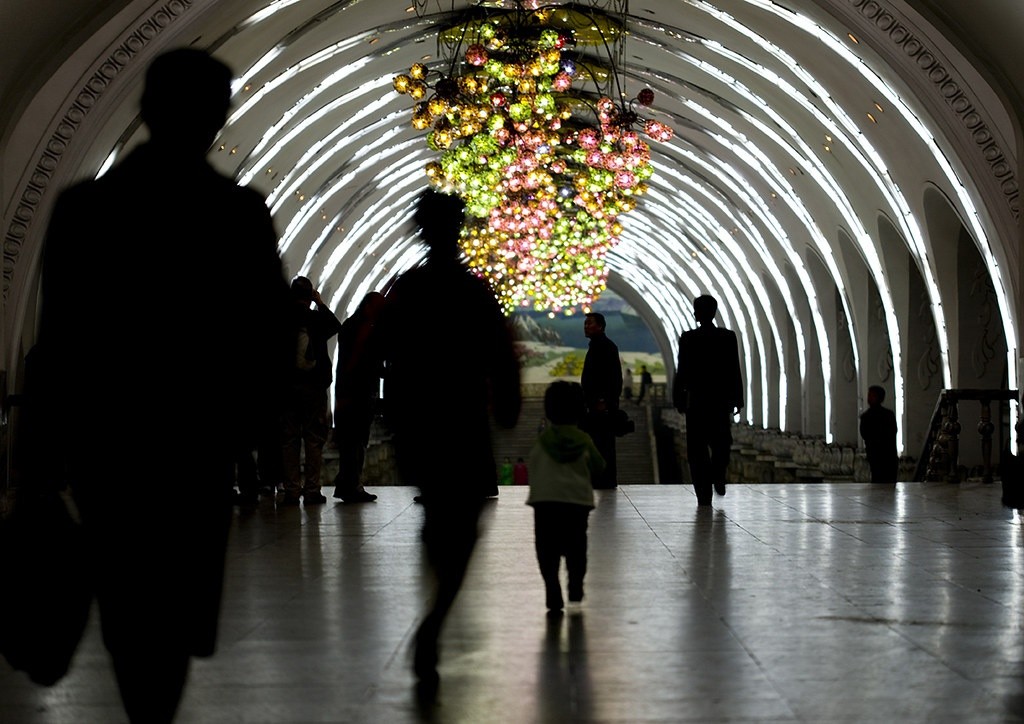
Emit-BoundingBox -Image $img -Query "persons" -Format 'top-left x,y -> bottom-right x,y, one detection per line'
673,293 -> 746,508
329,292 -> 386,506
633,364 -> 652,405
370,186 -> 518,711
859,384 -> 899,483
523,380 -> 603,616
578,312 -> 623,489
237,274 -> 341,517
622,368 -> 633,407
0,44 -> 297,722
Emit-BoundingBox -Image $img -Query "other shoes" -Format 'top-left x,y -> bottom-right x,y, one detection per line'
303,492 -> 327,507
236,498 -> 300,523
412,495 -> 421,504
697,496 -> 712,507
334,488 -> 379,506
546,587 -> 566,608
710,463 -> 727,496
566,579 -> 584,602
